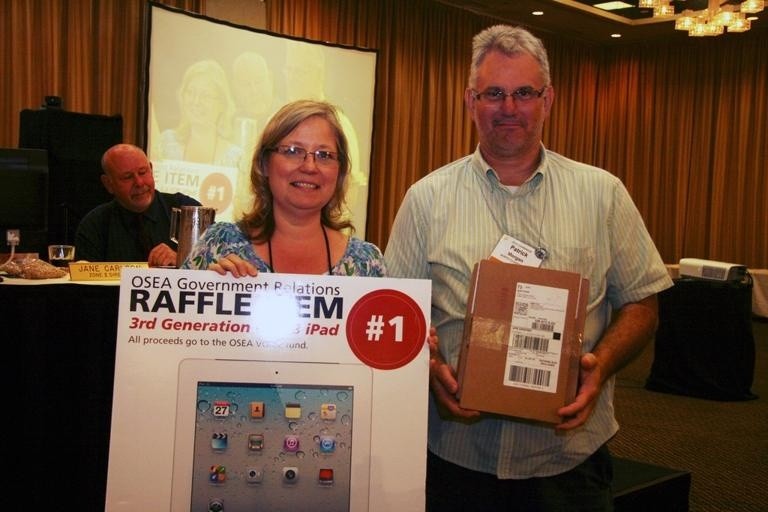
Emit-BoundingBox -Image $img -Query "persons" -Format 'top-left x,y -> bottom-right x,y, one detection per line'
178,99 -> 386,279
73,144 -> 204,266
383,23 -> 674,511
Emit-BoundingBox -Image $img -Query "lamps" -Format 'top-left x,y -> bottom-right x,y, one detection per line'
638,0 -> 764,38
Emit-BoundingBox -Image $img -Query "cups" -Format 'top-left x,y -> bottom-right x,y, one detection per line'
47,245 -> 75,266
170,205 -> 215,268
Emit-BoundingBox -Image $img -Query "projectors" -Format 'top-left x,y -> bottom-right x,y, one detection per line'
678,258 -> 748,282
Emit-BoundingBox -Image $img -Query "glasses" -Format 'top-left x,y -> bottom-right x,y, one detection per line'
472,86 -> 547,101
272,145 -> 342,165
